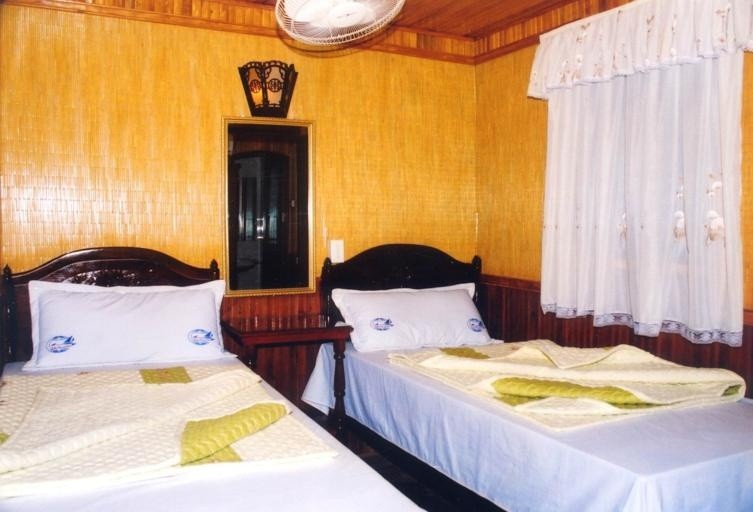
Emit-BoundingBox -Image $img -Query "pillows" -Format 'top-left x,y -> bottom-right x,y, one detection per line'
328,282 -> 503,352
20,278 -> 239,372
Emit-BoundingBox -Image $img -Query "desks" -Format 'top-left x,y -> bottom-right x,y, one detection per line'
223,314 -> 355,450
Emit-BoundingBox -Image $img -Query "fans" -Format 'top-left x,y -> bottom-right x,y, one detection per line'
271,0 -> 405,48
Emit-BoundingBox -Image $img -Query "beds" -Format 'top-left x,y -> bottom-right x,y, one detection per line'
320,243 -> 752,510
0,244 -> 428,512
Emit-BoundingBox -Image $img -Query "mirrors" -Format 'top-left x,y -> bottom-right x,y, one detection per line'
220,113 -> 317,299
